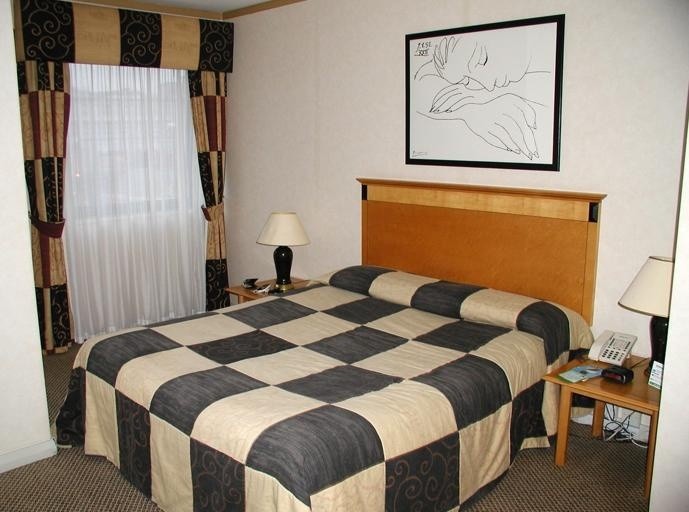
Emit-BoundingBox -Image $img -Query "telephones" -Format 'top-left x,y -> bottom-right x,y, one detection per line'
587,329 -> 638,366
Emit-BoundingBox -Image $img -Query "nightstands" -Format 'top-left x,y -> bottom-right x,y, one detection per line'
225,275 -> 320,303
540,353 -> 664,499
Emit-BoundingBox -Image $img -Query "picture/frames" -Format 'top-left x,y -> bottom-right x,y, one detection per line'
404,13 -> 568,173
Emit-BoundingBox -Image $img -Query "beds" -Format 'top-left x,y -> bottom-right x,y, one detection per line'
73,177 -> 608,512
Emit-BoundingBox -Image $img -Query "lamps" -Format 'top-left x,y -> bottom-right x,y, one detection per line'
257,210 -> 311,292
617,254 -> 675,376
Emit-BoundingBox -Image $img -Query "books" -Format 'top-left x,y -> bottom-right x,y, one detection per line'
555,365 -> 605,384
